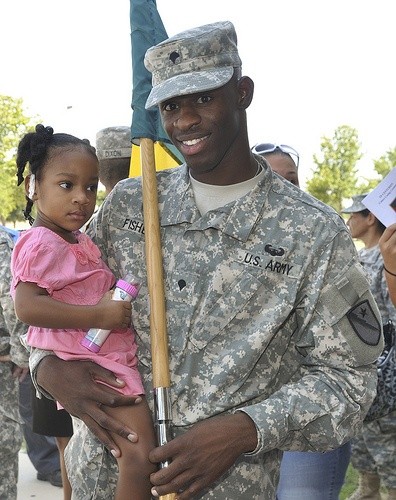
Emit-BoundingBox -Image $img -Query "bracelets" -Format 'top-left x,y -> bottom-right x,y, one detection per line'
382,265 -> 396,277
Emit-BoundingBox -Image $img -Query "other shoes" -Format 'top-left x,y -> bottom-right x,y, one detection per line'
37,470 -> 62,487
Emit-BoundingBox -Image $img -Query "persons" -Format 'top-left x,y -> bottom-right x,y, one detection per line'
342,193 -> 395,499
379,222 -> 396,306
78,126 -> 140,243
252,140 -> 355,500
29,376 -> 74,500
17,22 -> 385,499
8,122 -> 160,500
0,227 -> 32,500
18,355 -> 65,488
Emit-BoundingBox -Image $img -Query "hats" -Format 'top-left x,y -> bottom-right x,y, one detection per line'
143,21 -> 242,110
95,126 -> 132,160
340,194 -> 368,213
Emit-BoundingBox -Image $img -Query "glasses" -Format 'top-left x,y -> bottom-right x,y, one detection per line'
250,143 -> 300,170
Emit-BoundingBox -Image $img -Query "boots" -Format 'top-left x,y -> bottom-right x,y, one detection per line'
342,473 -> 381,500
